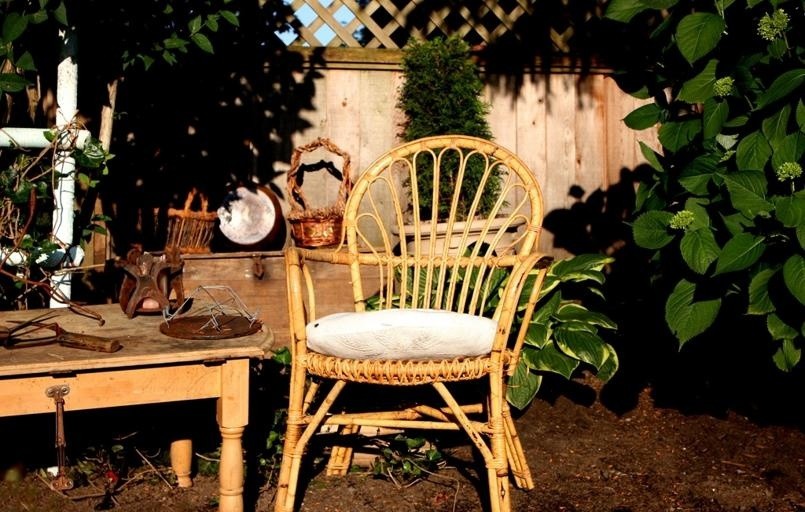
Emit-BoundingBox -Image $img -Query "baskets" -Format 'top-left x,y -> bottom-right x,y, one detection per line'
286,136 -> 352,248
139,186 -> 215,253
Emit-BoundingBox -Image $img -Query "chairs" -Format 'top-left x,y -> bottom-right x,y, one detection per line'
275,136 -> 554,512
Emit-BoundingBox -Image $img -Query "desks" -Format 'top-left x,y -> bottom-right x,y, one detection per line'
1,297 -> 274,512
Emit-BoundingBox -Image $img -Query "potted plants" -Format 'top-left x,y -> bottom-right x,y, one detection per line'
392,36 -> 526,267
